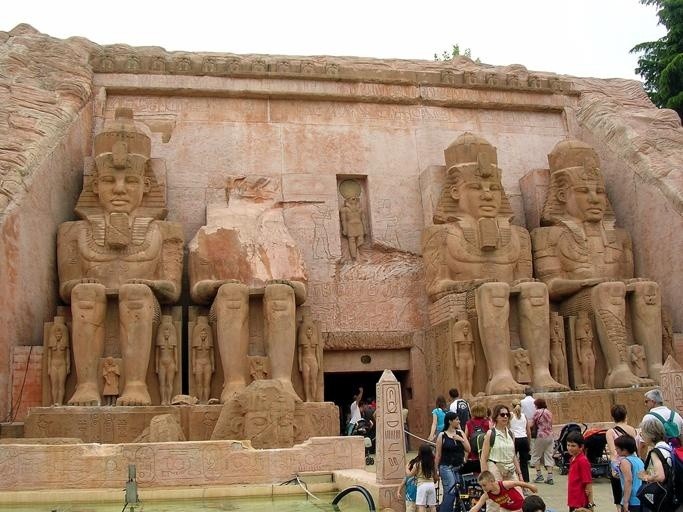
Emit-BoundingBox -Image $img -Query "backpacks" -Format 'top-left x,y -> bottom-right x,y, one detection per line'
469,420 -> 513,463
647,410 -> 680,438
654,442 -> 683,509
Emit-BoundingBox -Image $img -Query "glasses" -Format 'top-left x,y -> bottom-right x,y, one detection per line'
499,412 -> 511,418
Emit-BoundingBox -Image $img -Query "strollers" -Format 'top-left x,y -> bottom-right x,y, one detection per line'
347,417 -> 376,466
450,460 -> 488,511
579,428 -> 614,479
552,418 -> 587,476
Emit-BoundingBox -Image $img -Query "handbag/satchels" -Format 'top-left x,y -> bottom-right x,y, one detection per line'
494,463 -> 516,481
634,480 -> 670,512
530,422 -> 539,439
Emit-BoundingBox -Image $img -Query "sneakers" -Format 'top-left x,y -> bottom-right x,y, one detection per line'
533,473 -> 545,482
544,479 -> 555,485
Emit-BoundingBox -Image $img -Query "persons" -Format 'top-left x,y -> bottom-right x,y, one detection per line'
426,131 -> 664,397
47,110 -> 322,409
339,196 -> 367,260
345,388 -> 683,512
